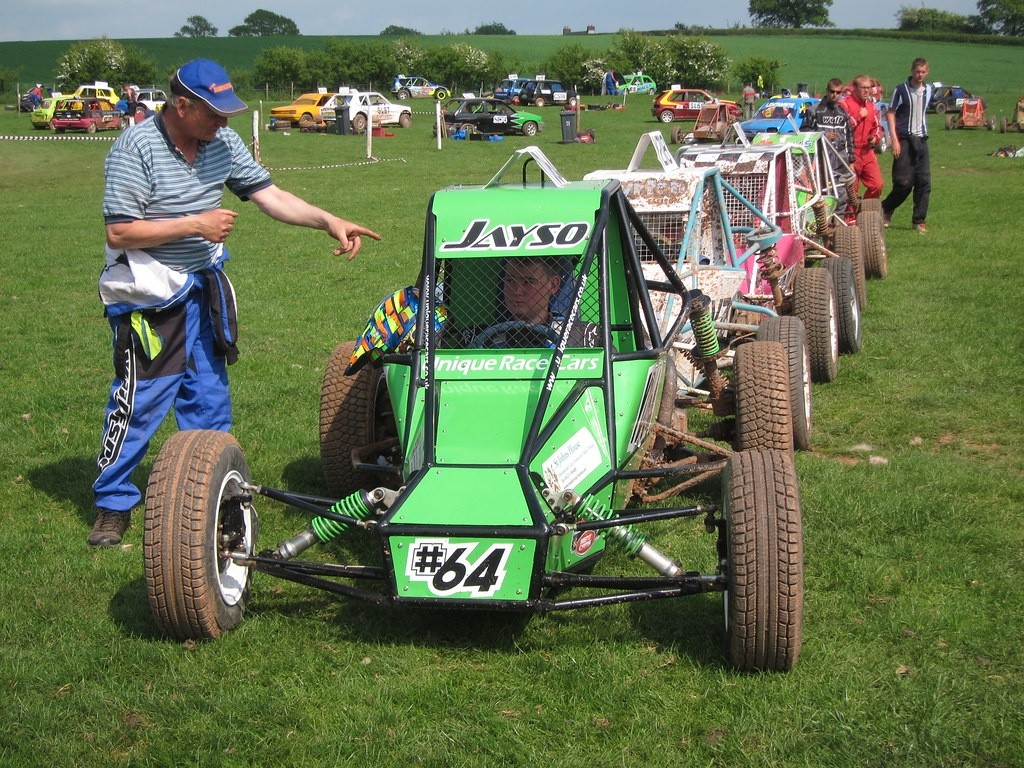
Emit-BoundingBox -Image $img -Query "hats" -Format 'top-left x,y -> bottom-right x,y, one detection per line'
171,61 -> 250,118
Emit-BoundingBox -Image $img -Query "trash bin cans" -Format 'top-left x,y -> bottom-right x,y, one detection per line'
797,82 -> 809,93
559,108 -> 576,140
333,105 -> 351,135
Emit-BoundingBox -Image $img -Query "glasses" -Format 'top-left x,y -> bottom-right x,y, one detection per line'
827,88 -> 842,94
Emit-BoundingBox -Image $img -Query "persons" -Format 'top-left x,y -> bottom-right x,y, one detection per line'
84,58 -> 382,546
741,81 -> 756,121
122,83 -> 136,128
449,254 -> 599,347
607,70 -> 617,95
813,59 -> 935,235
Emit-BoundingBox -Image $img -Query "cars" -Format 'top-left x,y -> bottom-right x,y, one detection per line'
60,85 -> 120,110
733,98 -> 821,144
31,97 -> 70,130
142,145 -> 804,674
391,77 -> 451,100
945,98 -> 1024,134
875,103 -> 892,153
433,98 -> 545,137
320,92 -> 412,130
927,87 -> 986,114
493,78 -> 535,106
518,80 -> 581,108
52,98 -> 128,134
612,71 -> 657,96
652,89 -> 743,123
674,114 -> 886,355
121,89 -> 168,114
20,87 -> 53,112
581,131 -> 837,451
270,93 -> 342,123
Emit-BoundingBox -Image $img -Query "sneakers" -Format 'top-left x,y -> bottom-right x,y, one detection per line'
86,505 -> 131,545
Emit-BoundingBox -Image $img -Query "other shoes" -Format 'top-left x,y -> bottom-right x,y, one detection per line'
913,221 -> 928,233
883,210 -> 893,228
